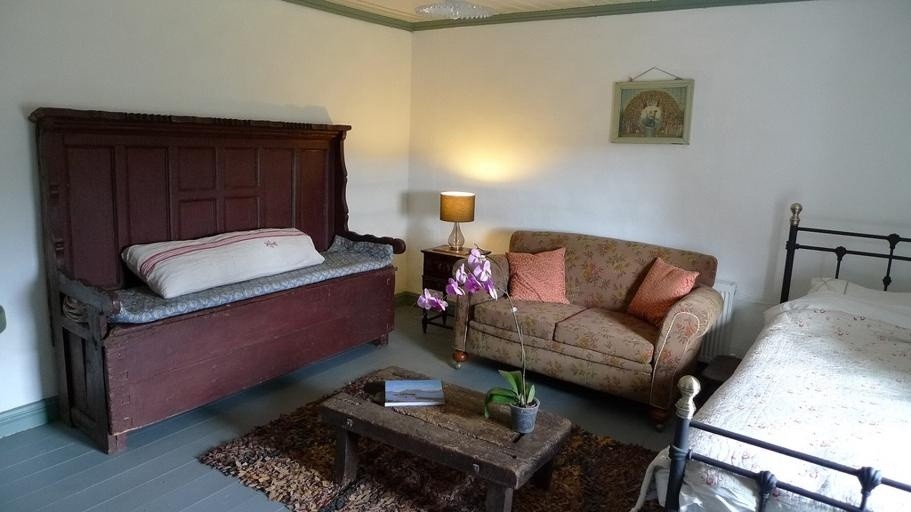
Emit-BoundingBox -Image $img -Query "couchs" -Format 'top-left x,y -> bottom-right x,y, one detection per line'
446,230 -> 728,433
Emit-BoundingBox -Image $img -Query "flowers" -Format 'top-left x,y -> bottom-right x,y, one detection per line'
413,243 -> 539,421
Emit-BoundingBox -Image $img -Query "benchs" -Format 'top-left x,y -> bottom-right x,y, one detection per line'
25,103 -> 408,457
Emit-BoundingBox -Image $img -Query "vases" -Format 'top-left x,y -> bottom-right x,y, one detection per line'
507,396 -> 539,435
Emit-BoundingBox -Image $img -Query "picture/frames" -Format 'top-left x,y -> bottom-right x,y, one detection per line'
610,80 -> 694,146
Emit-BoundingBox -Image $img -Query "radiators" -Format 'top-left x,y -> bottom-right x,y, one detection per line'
700,276 -> 740,364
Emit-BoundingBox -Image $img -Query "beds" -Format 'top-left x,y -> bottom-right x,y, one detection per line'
624,202 -> 911,512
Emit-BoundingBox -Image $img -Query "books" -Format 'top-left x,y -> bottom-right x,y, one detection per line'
384,379 -> 446,407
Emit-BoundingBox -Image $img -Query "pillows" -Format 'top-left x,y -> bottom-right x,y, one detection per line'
121,227 -> 327,300
503,247 -> 569,306
803,271 -> 910,311
624,255 -> 703,332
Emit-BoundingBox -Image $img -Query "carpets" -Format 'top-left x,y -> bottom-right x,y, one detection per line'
193,361 -> 671,512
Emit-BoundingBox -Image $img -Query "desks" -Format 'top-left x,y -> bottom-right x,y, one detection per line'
419,243 -> 493,336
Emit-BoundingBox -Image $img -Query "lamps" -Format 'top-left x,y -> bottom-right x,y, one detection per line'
435,188 -> 479,253
